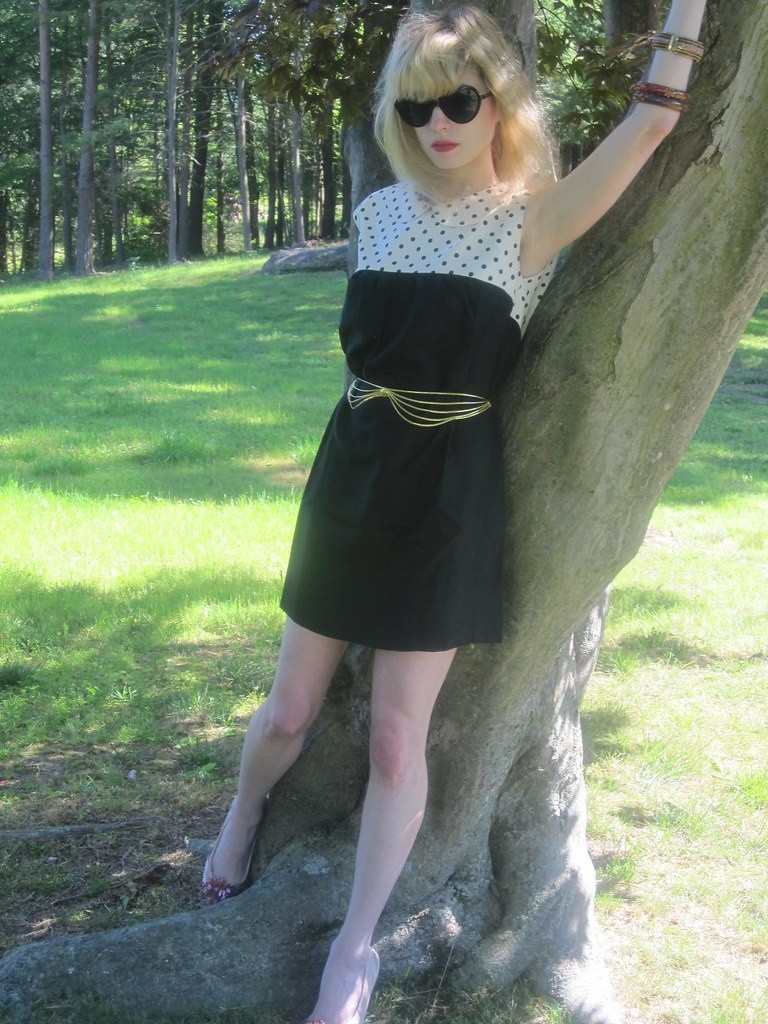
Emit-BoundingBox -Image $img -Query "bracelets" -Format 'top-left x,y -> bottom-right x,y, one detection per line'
635,81 -> 689,103
633,90 -> 689,115
650,32 -> 705,63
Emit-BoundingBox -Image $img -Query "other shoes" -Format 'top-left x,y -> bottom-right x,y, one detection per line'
302,943 -> 381,1024
201,795 -> 267,907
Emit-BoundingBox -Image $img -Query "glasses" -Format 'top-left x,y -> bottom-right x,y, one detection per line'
392,84 -> 492,128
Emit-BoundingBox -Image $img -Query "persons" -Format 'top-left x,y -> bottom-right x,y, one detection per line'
201,1 -> 703,1024
